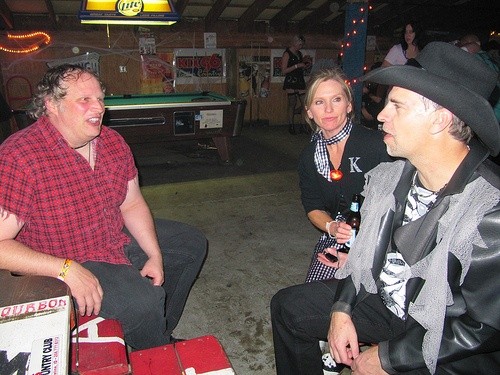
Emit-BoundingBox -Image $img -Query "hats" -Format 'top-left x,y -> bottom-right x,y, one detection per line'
361,40 -> 500,158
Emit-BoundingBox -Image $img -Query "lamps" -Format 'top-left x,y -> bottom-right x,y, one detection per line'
78,0 -> 180,25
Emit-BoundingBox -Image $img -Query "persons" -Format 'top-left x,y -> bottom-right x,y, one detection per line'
361,62 -> 388,123
0,62 -> 210,352
375,20 -> 431,111
281,35 -> 313,136
266,35 -> 500,375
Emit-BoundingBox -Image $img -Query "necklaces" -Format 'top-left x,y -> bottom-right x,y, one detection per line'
88,141 -> 91,164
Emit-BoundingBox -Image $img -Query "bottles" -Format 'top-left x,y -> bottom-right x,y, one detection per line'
259,67 -> 270,98
162,62 -> 174,93
344,192 -> 361,249
239,72 -> 250,101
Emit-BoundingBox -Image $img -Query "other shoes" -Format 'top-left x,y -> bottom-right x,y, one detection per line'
323,353 -> 344,375
289,128 -> 297,135
301,126 -> 309,134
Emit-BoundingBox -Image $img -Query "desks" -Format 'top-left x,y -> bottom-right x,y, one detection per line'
12,91 -> 246,166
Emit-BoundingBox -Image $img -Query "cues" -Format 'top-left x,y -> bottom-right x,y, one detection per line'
257,41 -> 260,120
250,41 -> 253,121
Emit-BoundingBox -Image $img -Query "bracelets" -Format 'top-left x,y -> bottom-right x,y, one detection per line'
294,64 -> 297,69
59,258 -> 72,280
326,219 -> 338,240
